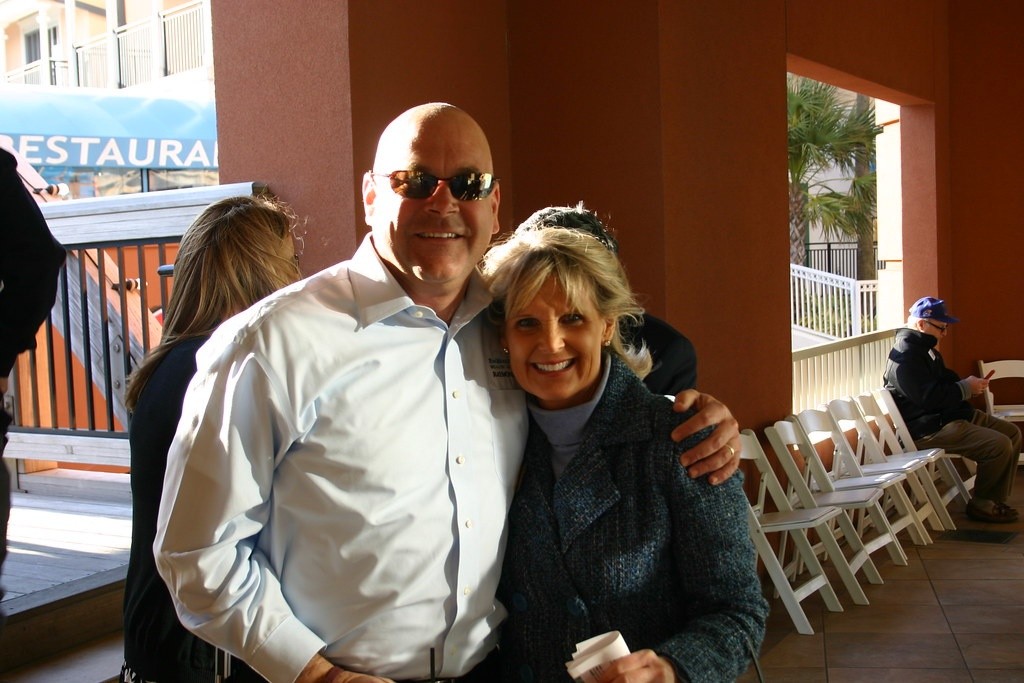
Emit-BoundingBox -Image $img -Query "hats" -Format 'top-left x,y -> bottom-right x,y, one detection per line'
909,296 -> 961,323
508,204 -> 619,256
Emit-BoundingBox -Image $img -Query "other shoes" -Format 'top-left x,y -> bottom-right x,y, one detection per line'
965,499 -> 1017,524
992,499 -> 1019,516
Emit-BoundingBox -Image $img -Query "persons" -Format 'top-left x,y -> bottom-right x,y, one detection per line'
119,193 -> 303,683
484,226 -> 770,683
0,149 -> 66,630
883,296 -> 1022,523
151,99 -> 741,683
516,201 -> 698,395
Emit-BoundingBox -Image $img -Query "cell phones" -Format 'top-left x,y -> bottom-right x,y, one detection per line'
982,369 -> 996,380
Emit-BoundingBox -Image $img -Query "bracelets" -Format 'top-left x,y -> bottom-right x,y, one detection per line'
317,666 -> 344,683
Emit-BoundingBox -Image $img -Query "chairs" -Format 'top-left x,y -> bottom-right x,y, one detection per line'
794,405 -> 925,567
882,387 -> 981,499
735,429 -> 843,635
767,416 -> 885,605
828,397 -> 925,558
980,360 -> 1024,465
849,391 -> 944,544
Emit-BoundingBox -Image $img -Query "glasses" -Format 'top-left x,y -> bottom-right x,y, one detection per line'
372,170 -> 501,202
923,320 -> 948,334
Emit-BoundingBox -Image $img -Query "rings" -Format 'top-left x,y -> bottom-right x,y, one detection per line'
724,444 -> 735,456
